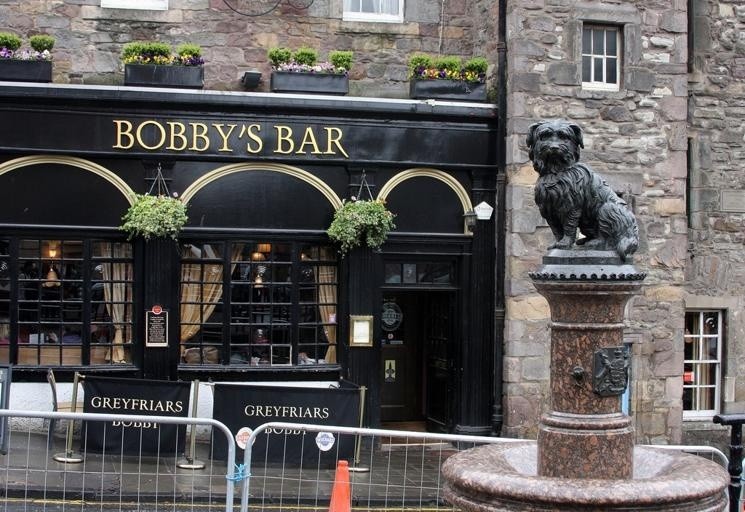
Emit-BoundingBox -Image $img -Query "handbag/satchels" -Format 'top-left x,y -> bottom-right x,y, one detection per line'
182,347 -> 219,364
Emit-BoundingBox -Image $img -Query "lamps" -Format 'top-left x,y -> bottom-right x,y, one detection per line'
41,237 -> 64,291
242,245 -> 271,289
241,70 -> 262,89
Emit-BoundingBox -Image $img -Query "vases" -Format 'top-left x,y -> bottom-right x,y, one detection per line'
1,63 -> 53,82
272,75 -> 349,97
124,66 -> 203,89
409,80 -> 488,101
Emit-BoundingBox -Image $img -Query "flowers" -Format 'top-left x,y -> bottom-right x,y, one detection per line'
265,42 -> 355,74
1,30 -> 57,60
118,37 -> 211,65
407,53 -> 488,79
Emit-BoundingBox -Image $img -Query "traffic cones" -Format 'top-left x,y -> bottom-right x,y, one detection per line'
327,459 -> 354,512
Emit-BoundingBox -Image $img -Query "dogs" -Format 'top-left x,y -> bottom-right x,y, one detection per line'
525,120 -> 640,265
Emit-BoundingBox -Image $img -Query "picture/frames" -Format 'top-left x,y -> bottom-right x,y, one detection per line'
144,307 -> 172,349
349,314 -> 375,348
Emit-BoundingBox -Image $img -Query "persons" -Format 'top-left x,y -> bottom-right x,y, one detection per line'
48,323 -> 81,345
0,316 -> 26,345
